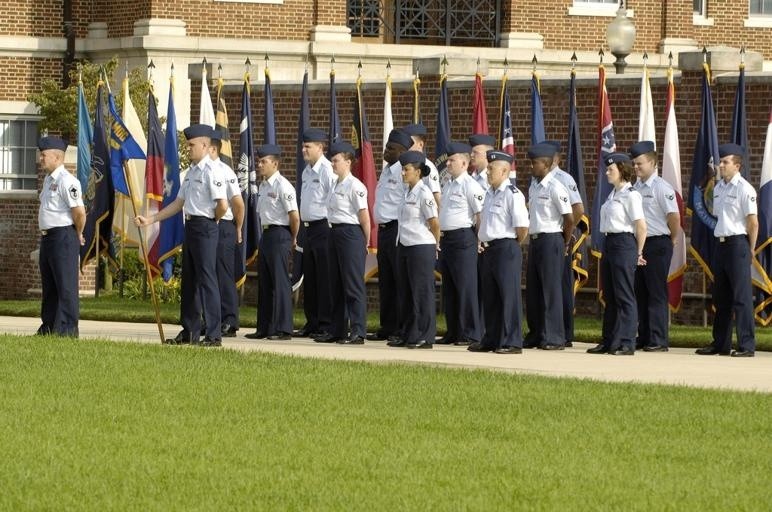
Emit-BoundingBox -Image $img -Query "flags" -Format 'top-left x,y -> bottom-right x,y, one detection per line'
751,107 -> 772,325
638,52 -> 657,151
145,60 -> 166,281
412,61 -> 422,124
157,63 -> 184,286
112,60 -> 148,270
103,68 -> 165,209
472,57 -> 488,136
234,57 -> 263,287
383,58 -> 393,170
215,63 -> 234,171
199,58 -> 216,129
76,72 -> 94,212
435,56 -> 452,173
565,51 -> 589,296
532,54 -> 546,146
662,52 -> 686,312
686,45 -> 722,282
292,54 -> 311,291
263,52 -> 276,144
731,46 -> 750,180
79,65 -> 115,274
327,53 -> 342,145
592,49 -> 619,308
351,60 -> 378,283
497,57 -> 516,186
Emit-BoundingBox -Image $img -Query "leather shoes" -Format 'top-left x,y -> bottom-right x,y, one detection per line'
643,343 -> 668,352
166,331 -> 192,344
199,338 -> 221,345
292,328 -> 314,338
222,322 -> 236,337
608,345 -> 633,355
522,342 -> 544,349
454,338 -> 473,345
437,336 -> 454,344
565,342 -> 572,347
336,335 -> 364,344
388,338 -> 406,347
267,331 -> 293,340
307,329 -> 331,338
543,342 -> 560,350
696,344 -> 730,355
587,345 -> 608,353
731,347 -> 754,356
314,333 -> 340,342
366,332 -> 385,341
495,346 -> 522,354
243,332 -> 269,339
637,341 -> 644,349
467,343 -> 496,352
407,340 -> 431,348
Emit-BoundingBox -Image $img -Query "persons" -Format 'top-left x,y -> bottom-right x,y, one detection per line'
367,128 -> 414,341
695,144 -> 759,358
585,153 -> 648,355
468,134 -> 496,191
526,145 -> 573,350
293,128 -> 338,338
528,140 -> 585,347
435,143 -> 486,346
316,143 -> 371,345
629,141 -> 681,351
405,124 -> 441,209
134,125 -> 228,348
468,151 -> 528,355
244,145 -> 300,340
35,137 -> 87,337
207,130 -> 244,338
387,151 -> 441,349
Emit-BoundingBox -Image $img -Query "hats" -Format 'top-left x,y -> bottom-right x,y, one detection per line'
212,130 -> 222,140
184,124 -> 213,140
303,128 -> 330,141
528,145 -> 556,158
330,143 -> 355,156
399,151 -> 425,166
539,141 -> 561,152
469,134 -> 497,147
403,124 -> 426,136
718,143 -> 745,158
389,130 -> 415,150
486,150 -> 514,165
604,152 -> 630,166
630,141 -> 654,157
37,137 -> 69,152
257,145 -> 282,158
445,142 -> 472,156
